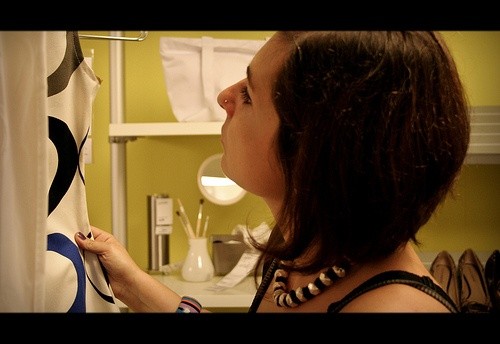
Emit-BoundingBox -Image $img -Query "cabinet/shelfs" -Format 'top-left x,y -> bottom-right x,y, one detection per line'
109,31 -> 261,313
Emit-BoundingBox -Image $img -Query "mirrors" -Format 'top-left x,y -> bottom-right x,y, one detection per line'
196,153 -> 247,206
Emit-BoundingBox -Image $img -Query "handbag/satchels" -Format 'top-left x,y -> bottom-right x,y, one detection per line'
161,35 -> 272,123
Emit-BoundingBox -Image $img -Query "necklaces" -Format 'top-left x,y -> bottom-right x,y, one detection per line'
273,248 -> 360,307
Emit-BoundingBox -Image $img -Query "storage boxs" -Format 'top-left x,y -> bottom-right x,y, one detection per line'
212,234 -> 263,275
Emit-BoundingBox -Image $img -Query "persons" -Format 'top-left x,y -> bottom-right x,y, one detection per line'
75,32 -> 471,313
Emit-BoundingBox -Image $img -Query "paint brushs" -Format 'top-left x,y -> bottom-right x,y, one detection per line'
196,198 -> 204,237
176,210 -> 190,238
177,197 -> 196,238
203,215 -> 209,237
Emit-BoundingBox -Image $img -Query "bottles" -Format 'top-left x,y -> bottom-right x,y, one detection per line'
181,236 -> 216,283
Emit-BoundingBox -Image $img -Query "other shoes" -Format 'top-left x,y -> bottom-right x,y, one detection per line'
484,250 -> 500,314
431,251 -> 458,307
458,249 -> 492,314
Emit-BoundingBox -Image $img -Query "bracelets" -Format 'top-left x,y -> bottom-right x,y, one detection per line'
176,296 -> 202,313
